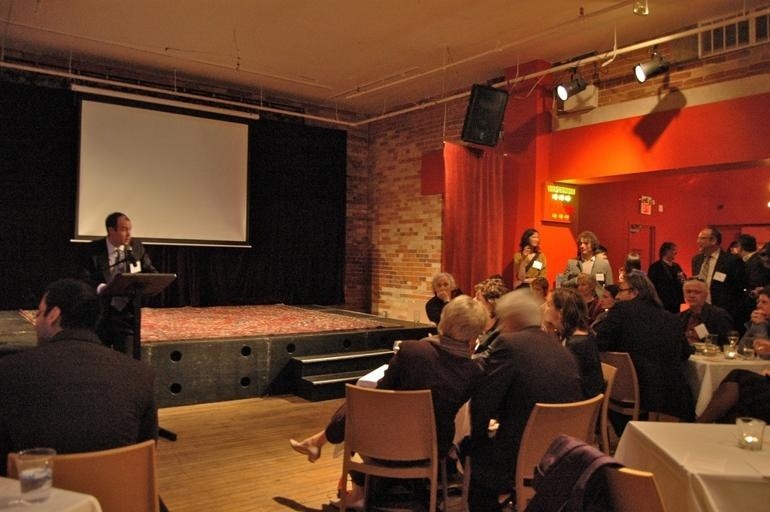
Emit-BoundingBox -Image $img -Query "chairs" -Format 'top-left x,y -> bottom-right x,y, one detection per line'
603,464 -> 665,512
461,393 -> 604,512
340,383 -> 447,512
594,362 -> 617,454
598,351 -> 643,421
8,438 -> 160,512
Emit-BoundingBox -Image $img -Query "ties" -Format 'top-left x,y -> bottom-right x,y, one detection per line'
114,249 -> 125,310
700,256 -> 711,281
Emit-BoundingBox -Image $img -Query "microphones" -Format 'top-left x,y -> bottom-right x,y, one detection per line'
122,243 -> 140,270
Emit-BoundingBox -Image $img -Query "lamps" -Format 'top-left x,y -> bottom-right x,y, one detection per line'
633,43 -> 669,84
555,67 -> 587,101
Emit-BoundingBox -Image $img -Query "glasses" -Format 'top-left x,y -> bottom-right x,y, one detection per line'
617,286 -> 631,293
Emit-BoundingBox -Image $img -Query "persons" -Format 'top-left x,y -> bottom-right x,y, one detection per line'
649,241 -> 686,312
77,211 -> 159,351
592,284 -> 620,332
288,295 -> 490,511
472,279 -> 509,353
576,271 -> 606,330
561,230 -> 613,288
694,337 -> 770,425
737,287 -> 770,353
510,228 -> 547,288
596,271 -> 697,437
622,251 -> 641,272
459,292 -> 582,512
424,272 -> 465,325
592,243 -> 609,261
0,278 -> 159,479
530,277 -> 552,313
736,234 -> 770,287
543,287 -> 605,399
691,228 -> 748,311
725,240 -> 738,253
671,278 -> 732,362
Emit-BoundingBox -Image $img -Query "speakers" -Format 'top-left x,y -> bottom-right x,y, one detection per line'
460,83 -> 510,148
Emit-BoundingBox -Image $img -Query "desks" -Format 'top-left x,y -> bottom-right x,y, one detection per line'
613,421 -> 770,512
686,355 -> 770,418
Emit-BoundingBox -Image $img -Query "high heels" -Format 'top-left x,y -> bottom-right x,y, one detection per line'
289,438 -> 321,463
498,490 -> 516,508
329,498 -> 364,509
